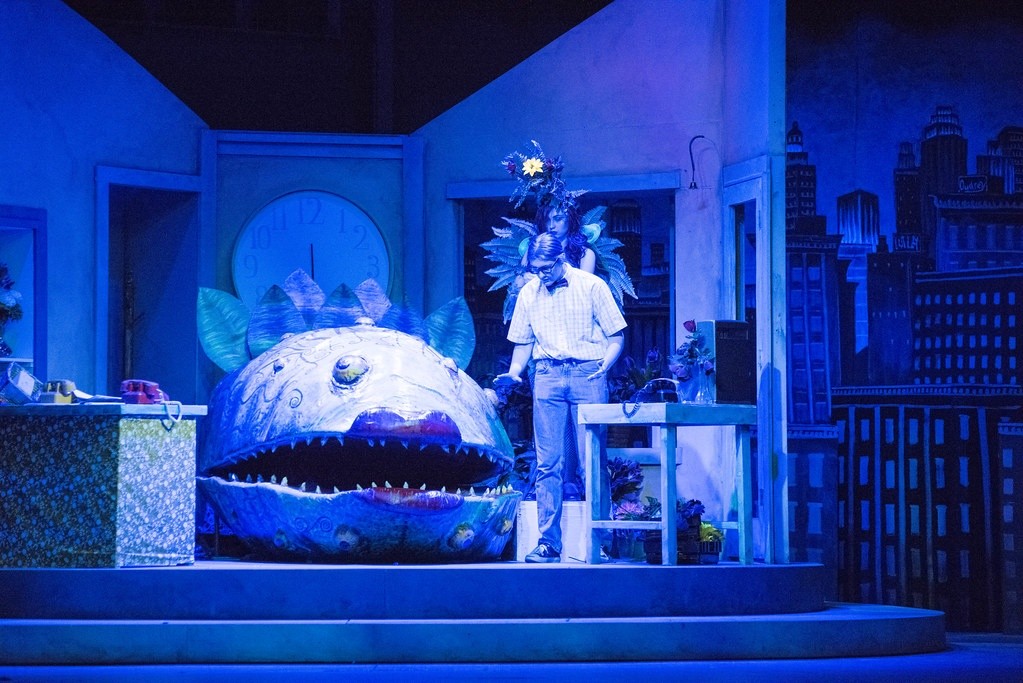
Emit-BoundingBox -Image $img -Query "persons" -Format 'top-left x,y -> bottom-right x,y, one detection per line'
479,189 -> 638,502
493,232 -> 627,563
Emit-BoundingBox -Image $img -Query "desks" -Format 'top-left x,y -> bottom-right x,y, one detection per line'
577,403 -> 759,567
0,403 -> 208,568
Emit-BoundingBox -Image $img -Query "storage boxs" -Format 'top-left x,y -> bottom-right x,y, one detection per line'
516,500 -> 588,563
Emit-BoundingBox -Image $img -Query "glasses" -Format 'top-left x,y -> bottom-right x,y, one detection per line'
527,258 -> 559,274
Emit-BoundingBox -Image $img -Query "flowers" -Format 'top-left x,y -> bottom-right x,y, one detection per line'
606,457 -> 705,532
699,522 -> 726,542
499,141 -> 574,211
668,319 -> 716,382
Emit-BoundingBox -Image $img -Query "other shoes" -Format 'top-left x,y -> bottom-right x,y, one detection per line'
563,483 -> 579,501
526,488 -> 535,501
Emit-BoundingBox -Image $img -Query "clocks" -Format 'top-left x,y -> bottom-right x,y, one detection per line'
229,188 -> 395,312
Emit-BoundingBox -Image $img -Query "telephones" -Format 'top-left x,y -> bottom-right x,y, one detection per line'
629,379 -> 679,403
37,379 -> 76,404
119,379 -> 169,405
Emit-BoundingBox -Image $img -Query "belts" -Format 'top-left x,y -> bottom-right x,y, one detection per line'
538,358 -> 590,364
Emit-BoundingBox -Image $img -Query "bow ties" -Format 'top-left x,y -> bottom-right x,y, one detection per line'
546,278 -> 568,292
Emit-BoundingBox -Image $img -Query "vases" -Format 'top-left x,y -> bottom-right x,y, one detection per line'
614,532 -> 723,565
695,366 -> 714,404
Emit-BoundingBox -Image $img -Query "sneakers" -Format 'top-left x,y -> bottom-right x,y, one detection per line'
584,546 -> 609,563
525,543 -> 561,563
